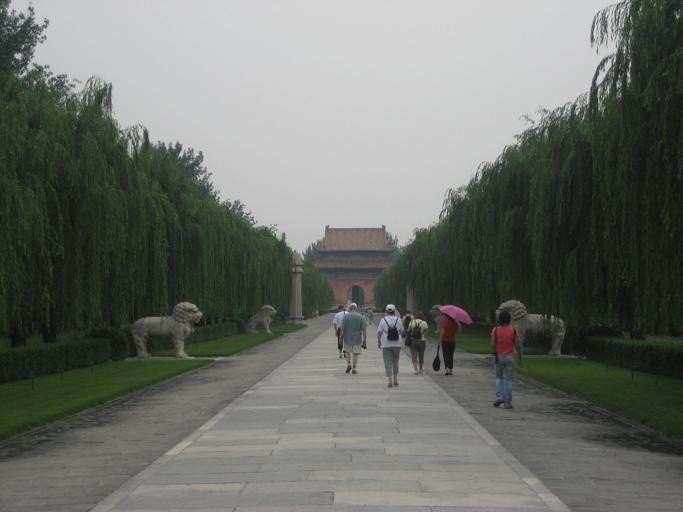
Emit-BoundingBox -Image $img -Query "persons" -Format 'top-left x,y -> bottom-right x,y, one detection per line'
491,311 -> 525,409
346,304 -> 416,347
338,302 -> 369,374
333,303 -> 347,359
406,311 -> 429,375
377,303 -> 406,387
439,313 -> 459,376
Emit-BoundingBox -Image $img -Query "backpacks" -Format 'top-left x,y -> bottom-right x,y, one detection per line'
403,315 -> 411,328
384,316 -> 398,340
412,319 -> 425,339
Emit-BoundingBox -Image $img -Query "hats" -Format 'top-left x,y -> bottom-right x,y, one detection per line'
385,304 -> 395,313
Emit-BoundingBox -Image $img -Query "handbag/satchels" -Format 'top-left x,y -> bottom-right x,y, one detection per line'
432,345 -> 440,371
404,326 -> 412,346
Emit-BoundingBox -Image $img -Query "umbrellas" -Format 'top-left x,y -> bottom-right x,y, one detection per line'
437,305 -> 473,326
